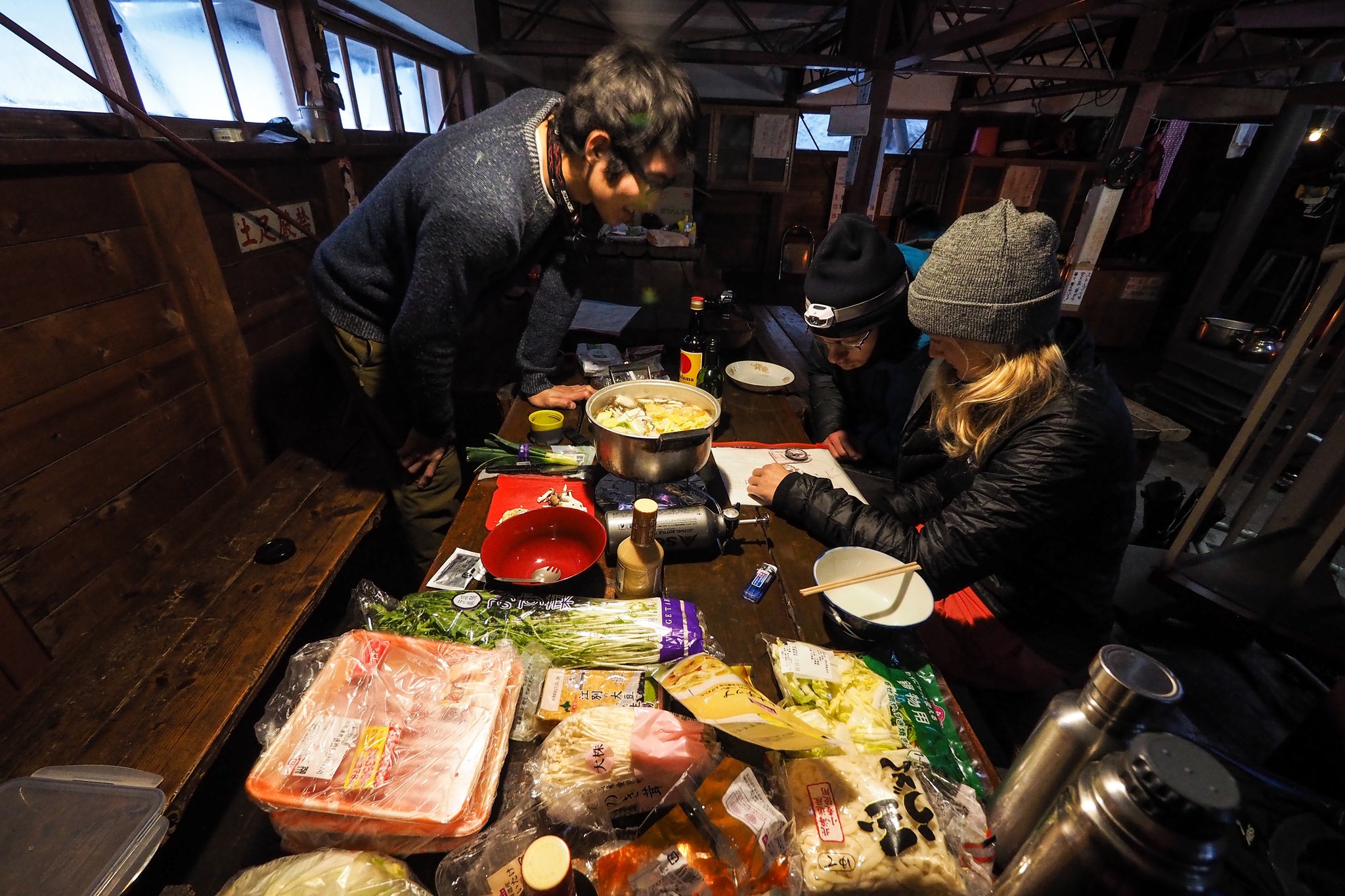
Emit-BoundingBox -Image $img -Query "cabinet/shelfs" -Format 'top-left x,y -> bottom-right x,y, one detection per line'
941,155 -> 1095,253
692,103 -> 800,193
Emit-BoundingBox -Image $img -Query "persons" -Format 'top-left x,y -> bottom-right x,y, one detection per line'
313,42 -> 701,585
806,212 -> 931,498
749,199 -> 1135,766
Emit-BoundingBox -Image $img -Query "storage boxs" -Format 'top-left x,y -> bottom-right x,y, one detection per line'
632,172 -> 693,228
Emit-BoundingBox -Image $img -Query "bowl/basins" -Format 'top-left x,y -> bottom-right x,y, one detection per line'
813,546 -> 934,642
1000,139 -> 1032,152
725,360 -> 795,392
481,507 -> 607,589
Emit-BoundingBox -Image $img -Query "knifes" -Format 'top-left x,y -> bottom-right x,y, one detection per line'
485,464 -> 599,473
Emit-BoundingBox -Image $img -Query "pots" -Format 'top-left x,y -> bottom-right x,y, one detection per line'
1195,317 -> 1269,350
585,363 -> 721,485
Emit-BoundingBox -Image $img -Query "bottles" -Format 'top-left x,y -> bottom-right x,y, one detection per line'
989,643 -> 1183,878
991,730 -> 1242,896
614,498 -> 664,600
521,834 -> 578,896
696,340 -> 724,441
679,296 -> 705,387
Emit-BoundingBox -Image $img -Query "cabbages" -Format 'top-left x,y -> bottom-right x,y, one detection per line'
772,638 -> 904,756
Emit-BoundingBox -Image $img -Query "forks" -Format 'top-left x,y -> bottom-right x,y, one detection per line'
494,566 -> 561,583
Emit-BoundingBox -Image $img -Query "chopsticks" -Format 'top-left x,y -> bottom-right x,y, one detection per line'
799,560 -> 922,596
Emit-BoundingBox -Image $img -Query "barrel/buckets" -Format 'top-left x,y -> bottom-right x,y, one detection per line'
970,127 -> 999,157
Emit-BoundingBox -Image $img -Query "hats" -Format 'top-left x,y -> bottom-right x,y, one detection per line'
908,200 -> 1062,342
803,213 -> 916,339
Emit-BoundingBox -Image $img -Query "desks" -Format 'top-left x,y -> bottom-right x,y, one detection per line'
416,261 -> 1000,896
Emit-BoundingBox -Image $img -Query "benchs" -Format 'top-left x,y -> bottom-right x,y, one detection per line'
1125,397 -> 1192,483
735,300 -> 826,395
0,451 -> 395,896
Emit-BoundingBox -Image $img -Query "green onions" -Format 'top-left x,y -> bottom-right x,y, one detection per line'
465,434 -> 578,473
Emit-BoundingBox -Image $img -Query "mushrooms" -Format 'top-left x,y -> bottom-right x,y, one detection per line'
537,483 -> 587,512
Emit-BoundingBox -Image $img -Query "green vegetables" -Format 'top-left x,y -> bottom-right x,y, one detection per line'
365,588 -> 707,666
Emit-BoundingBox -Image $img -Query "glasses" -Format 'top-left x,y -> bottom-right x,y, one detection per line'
806,326 -> 873,352
613,143 -> 673,201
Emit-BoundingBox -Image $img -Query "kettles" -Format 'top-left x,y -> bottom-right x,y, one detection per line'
1233,323 -> 1283,364
